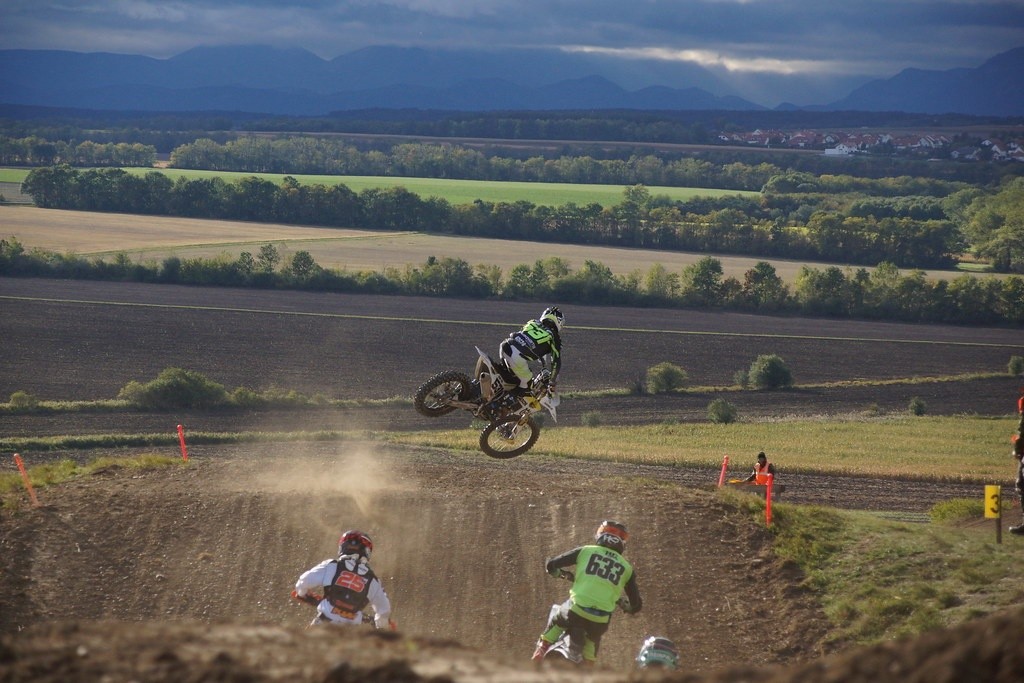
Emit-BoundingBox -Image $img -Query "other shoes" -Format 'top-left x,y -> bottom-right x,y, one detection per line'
1009,524 -> 1024,536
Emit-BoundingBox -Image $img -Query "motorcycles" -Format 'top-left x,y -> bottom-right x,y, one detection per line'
414,345 -> 557,459
290,588 -> 397,640
537,571 -> 628,677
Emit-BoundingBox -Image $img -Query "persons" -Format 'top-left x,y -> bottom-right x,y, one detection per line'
532,520 -> 643,668
296,529 -> 397,633
636,637 -> 678,670
499,306 -> 565,412
1010,397 -> 1024,536
743,452 -> 775,485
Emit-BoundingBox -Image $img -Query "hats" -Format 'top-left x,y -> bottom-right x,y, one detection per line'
758,452 -> 765,458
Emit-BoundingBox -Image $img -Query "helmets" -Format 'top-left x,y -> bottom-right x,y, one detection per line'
540,307 -> 564,332
596,520 -> 629,551
638,637 -> 676,671
338,531 -> 373,563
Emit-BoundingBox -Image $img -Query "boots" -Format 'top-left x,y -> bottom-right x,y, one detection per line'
531,640 -> 549,663
579,659 -> 592,673
